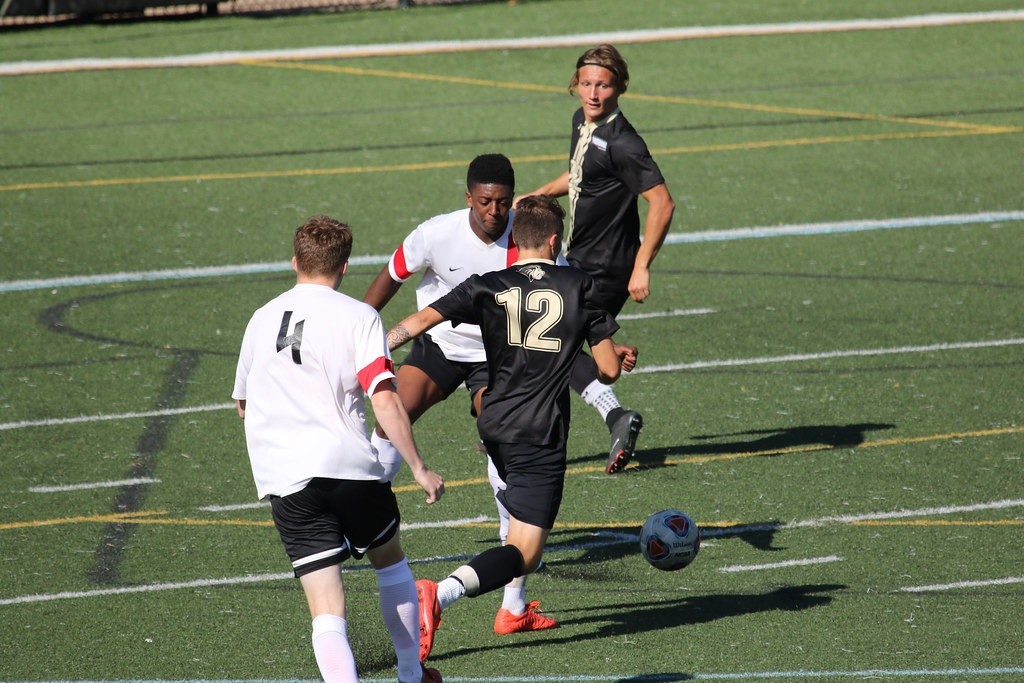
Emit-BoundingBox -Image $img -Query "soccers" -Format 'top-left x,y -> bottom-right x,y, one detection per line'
639,508 -> 701,572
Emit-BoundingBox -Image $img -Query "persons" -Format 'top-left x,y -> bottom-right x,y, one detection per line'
364,154 -> 569,481
514,44 -> 677,475
230,217 -> 445,683
387,194 -> 621,661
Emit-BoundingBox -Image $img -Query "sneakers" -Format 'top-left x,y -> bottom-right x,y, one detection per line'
416,580 -> 442,661
606,412 -> 645,474
494,600 -> 557,634
398,661 -> 442,683
476,442 -> 487,451
